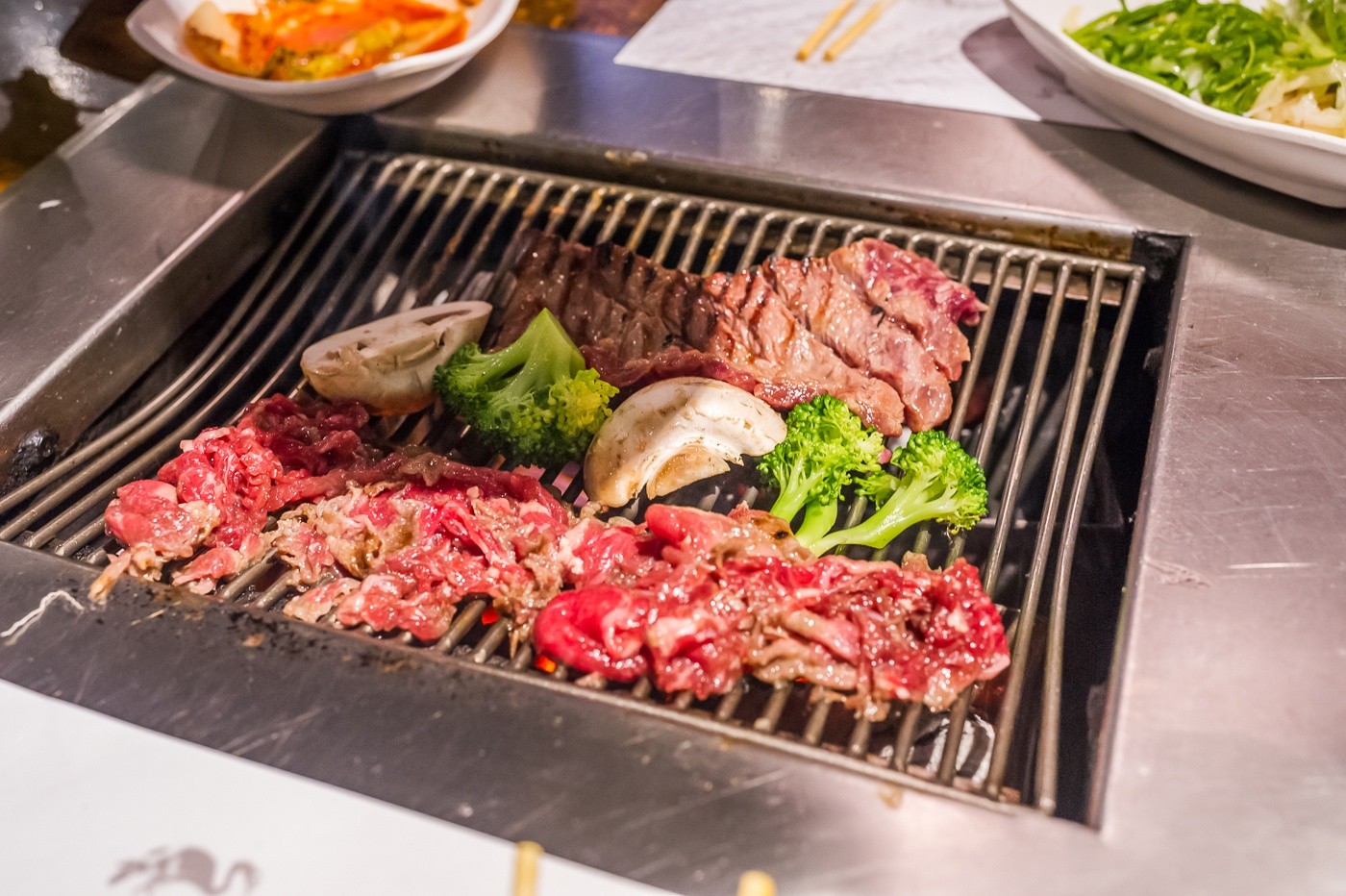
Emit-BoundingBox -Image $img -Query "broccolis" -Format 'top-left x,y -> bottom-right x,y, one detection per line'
756,392 -> 988,558
431,307 -> 619,466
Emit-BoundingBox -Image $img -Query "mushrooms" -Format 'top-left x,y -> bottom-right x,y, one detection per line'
581,374 -> 788,509
299,299 -> 490,418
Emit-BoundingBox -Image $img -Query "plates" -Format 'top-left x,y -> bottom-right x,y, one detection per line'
124,1 -> 522,113
1006,0 -> 1345,209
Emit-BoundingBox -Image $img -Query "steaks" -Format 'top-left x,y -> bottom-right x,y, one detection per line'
485,229 -> 987,436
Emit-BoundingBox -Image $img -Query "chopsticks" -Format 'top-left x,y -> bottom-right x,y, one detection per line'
796,0 -> 896,61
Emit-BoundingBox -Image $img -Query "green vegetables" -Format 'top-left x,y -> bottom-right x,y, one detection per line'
1065,0 -> 1346,134
